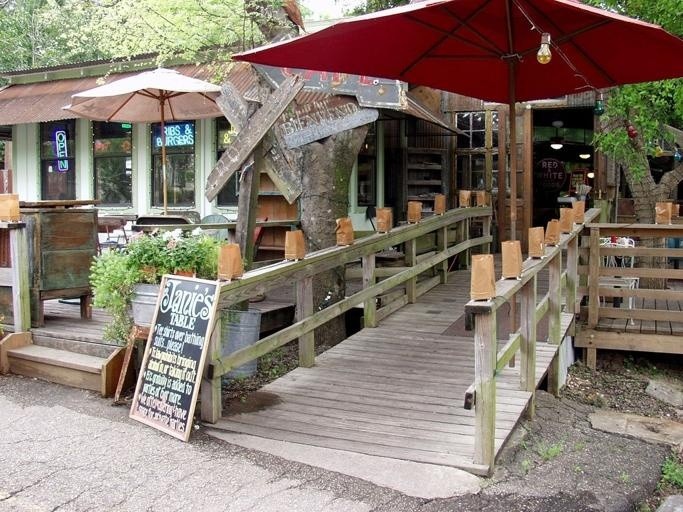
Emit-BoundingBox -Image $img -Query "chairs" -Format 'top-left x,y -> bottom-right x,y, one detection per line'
593,237 -> 639,325
99,210 -> 231,245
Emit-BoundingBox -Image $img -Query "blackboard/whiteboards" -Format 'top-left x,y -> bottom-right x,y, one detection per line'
569,170 -> 586,192
129,274 -> 222,443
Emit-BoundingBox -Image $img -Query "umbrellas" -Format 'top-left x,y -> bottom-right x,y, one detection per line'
61,65 -> 227,214
229,0 -> 682,244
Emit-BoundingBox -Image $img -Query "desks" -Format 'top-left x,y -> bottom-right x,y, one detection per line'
113,326 -> 150,401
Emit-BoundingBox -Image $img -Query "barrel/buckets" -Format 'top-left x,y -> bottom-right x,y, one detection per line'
222,307 -> 261,382
128,283 -> 160,327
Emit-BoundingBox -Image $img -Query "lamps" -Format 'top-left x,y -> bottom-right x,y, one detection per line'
549,126 -> 562,149
536,32 -> 553,65
653,138 -> 663,157
672,145 -> 682,161
578,129 -> 591,160
622,119 -> 638,137
593,91 -> 604,112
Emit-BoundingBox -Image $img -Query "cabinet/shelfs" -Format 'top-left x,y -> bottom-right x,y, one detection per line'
401,146 -> 451,219
0,200 -> 97,326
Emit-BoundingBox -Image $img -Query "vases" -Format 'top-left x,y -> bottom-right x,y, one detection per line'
127,265 -> 197,326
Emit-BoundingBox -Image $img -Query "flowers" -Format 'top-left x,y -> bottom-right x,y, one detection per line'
90,231 -> 223,311
